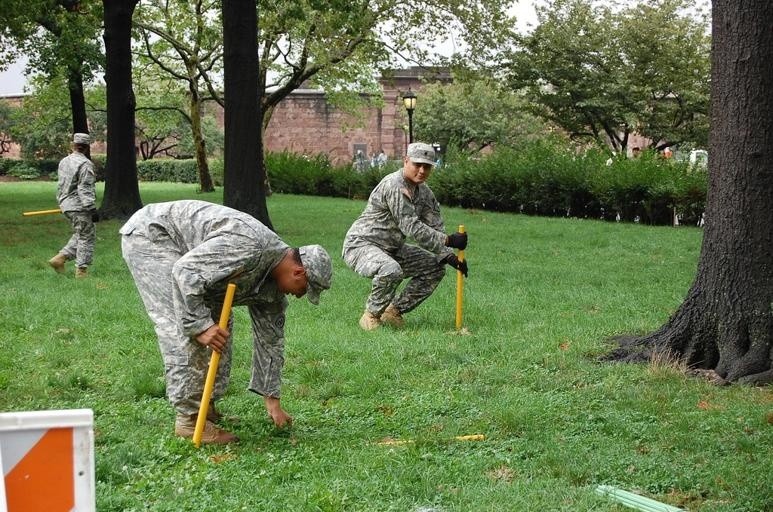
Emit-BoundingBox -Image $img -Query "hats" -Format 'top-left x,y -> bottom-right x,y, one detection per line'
407,143 -> 435,166
74,133 -> 90,144
299,245 -> 332,305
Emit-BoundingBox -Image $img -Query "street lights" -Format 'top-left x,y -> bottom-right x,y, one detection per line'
403,86 -> 417,143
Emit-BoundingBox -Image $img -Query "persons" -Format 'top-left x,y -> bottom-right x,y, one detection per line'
629,147 -> 640,160
340,143 -> 468,332
329,147 -> 387,176
116,199 -> 334,443
48,132 -> 99,279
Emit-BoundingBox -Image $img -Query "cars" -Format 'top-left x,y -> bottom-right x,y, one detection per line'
678,149 -> 708,177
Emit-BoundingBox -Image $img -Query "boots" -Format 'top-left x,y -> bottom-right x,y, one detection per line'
75,268 -> 88,277
49,253 -> 65,273
359,309 -> 382,329
175,398 -> 238,444
380,302 -> 403,324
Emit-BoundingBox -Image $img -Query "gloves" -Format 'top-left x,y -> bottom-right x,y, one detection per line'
91,208 -> 99,222
446,254 -> 468,277
448,231 -> 467,250
